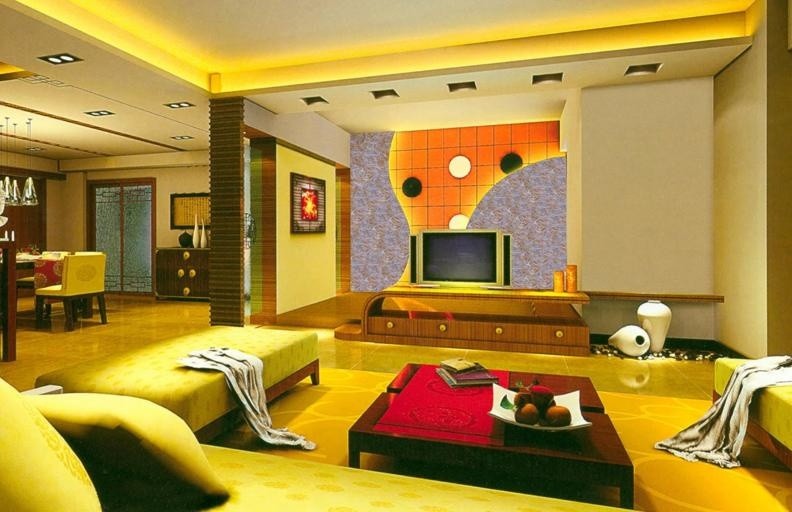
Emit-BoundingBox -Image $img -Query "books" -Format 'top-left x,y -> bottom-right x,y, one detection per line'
434,357 -> 499,390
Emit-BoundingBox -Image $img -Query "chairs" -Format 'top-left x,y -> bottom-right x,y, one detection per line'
17,250 -> 107,331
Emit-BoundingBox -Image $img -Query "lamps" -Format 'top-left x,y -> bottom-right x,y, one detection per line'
0,117 -> 39,206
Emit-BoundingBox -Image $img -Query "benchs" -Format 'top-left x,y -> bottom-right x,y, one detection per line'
19,384 -> 633,511
34,326 -> 319,441
712,356 -> 791,467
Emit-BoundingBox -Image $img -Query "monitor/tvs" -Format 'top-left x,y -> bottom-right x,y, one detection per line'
410,229 -> 514,290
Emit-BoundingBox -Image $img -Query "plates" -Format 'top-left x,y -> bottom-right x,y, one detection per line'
486,382 -> 592,433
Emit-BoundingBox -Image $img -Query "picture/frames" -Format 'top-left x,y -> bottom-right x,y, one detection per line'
290,172 -> 326,234
170,192 -> 211,229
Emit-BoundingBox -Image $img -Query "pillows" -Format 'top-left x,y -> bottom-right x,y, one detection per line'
32,392 -> 229,502
0,377 -> 102,512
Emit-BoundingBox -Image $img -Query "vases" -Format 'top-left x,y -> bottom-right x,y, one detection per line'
192,215 -> 200,248
200,220 -> 208,249
637,300 -> 672,353
608,325 -> 651,357
179,230 -> 193,248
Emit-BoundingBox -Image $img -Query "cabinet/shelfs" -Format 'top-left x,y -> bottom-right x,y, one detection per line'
154,248 -> 210,301
334,292 -> 592,356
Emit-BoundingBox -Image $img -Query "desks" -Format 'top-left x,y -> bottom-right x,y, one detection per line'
0,241 -> 17,361
